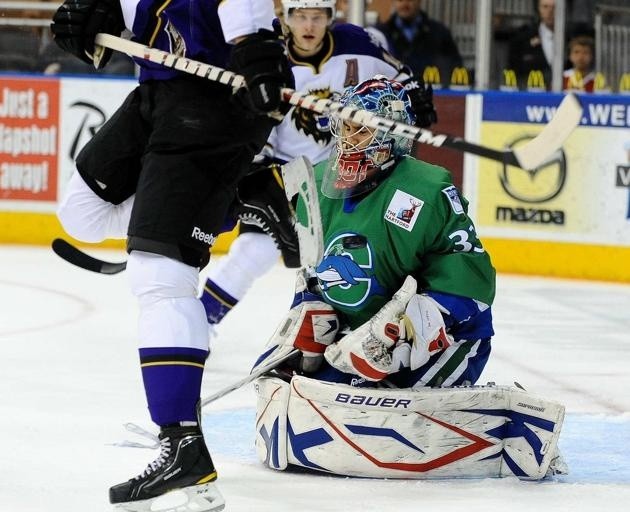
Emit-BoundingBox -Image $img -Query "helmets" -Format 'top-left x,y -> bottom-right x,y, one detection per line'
320,75 -> 417,200
281,0 -> 336,27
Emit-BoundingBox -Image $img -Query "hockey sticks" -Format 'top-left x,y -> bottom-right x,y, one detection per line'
52,239 -> 126,275
295,156 -> 324,265
96,32 -> 584,170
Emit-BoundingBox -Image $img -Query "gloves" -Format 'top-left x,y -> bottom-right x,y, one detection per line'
226,28 -> 296,126
50,0 -> 126,71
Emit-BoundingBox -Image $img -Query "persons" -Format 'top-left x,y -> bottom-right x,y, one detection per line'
49,0 -> 325,512
378,1 -> 466,86
489,2 -> 610,94
198,0 -> 438,329
250,76 -> 569,482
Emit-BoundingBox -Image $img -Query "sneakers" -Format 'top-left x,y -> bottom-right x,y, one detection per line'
241,163 -> 302,268
109,421 -> 217,503
